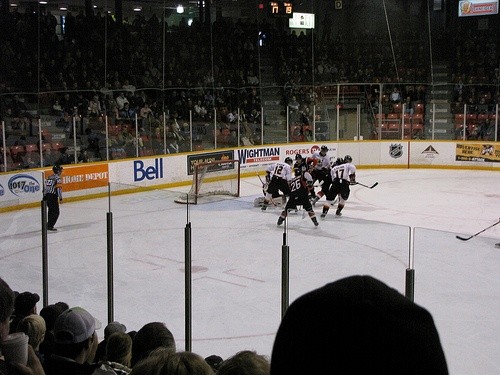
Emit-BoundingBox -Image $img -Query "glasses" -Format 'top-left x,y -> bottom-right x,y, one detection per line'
7,314 -> 16,323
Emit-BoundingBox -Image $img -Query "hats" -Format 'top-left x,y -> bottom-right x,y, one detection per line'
51,306 -> 103,345
104,322 -> 126,339
270,274 -> 450,375
14,292 -> 40,311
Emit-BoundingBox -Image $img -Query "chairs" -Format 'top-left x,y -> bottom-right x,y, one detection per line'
0,0 -> 500,176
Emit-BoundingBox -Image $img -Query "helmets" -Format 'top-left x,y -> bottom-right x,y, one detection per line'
53,164 -> 64,174
285,145 -> 352,176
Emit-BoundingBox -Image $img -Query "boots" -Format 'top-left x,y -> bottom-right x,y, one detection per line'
261,194 -> 345,228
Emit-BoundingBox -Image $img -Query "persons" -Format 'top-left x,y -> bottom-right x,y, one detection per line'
0,275 -> 451,375
0,7 -> 500,247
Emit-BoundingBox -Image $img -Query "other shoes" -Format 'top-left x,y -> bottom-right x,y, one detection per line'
48,228 -> 57,232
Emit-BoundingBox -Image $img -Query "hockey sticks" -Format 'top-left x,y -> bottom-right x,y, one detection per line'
356,182 -> 378,189
456,220 -> 500,241
257,172 -> 281,208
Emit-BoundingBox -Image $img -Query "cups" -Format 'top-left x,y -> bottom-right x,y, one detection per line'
0,333 -> 29,367
